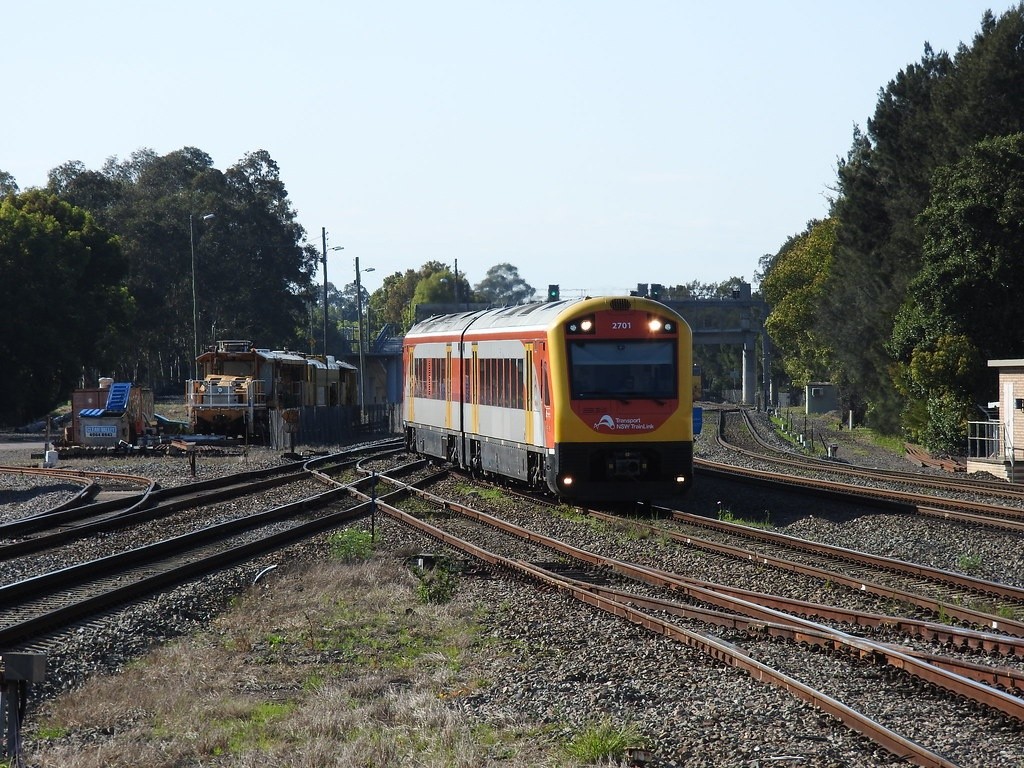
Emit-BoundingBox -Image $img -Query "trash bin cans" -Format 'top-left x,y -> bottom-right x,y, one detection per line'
76,382 -> 132,447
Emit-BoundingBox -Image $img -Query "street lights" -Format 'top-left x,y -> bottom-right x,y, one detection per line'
320,227 -> 345,355
189,213 -> 216,380
354,257 -> 378,424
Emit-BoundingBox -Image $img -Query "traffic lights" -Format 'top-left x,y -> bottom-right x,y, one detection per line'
548,284 -> 560,303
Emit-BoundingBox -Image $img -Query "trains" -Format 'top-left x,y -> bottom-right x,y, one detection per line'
183,340 -> 357,438
402,296 -> 694,503
